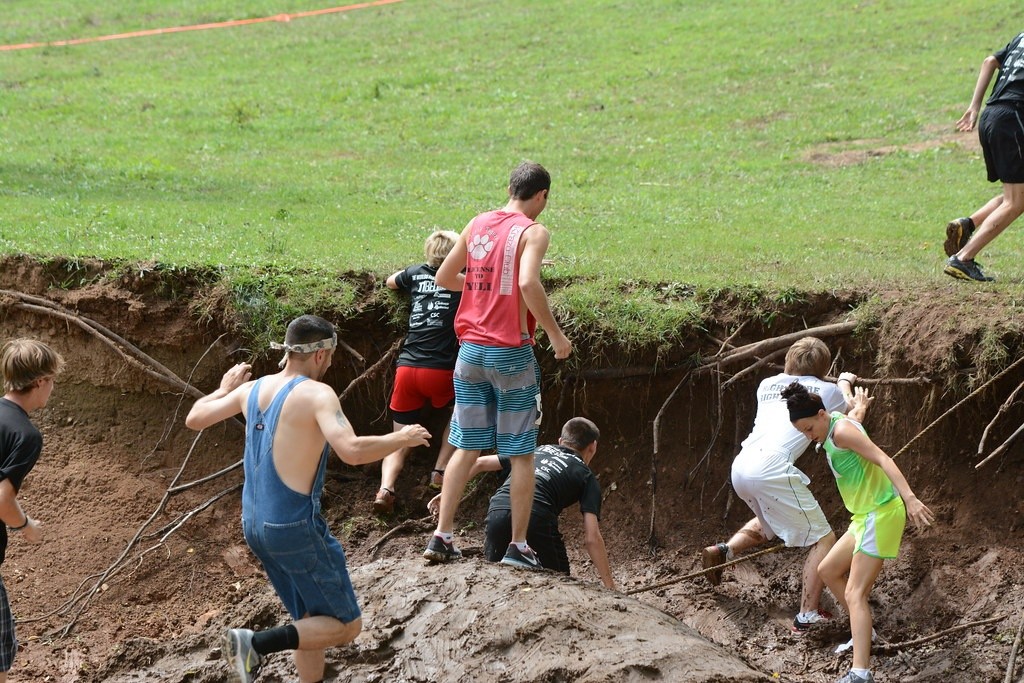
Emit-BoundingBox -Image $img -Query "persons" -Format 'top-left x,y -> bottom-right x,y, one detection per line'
427,417 -> 616,591
779,381 -> 935,683
0,337 -> 67,683
185,314 -> 433,683
943,31 -> 1024,282
702,337 -> 858,636
423,162 -> 571,572
373,230 -> 554,515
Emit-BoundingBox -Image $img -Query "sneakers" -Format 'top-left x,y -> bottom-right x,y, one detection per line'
837,670 -> 874,683
221,628 -> 264,683
702,543 -> 728,586
945,218 -> 970,257
944,253 -> 995,284
423,535 -> 462,560
791,609 -> 830,634
501,543 -> 544,571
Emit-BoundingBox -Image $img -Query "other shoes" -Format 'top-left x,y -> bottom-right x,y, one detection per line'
374,489 -> 395,515
430,471 -> 442,490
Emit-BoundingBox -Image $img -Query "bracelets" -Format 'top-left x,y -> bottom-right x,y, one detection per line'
837,378 -> 851,385
8,517 -> 28,531
